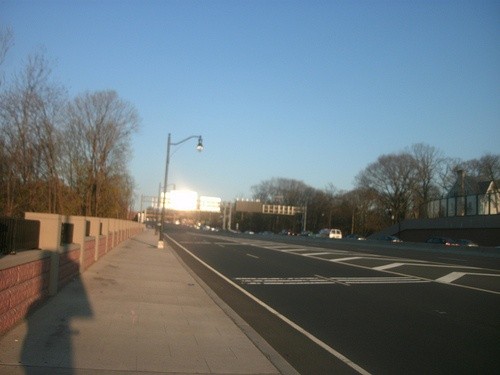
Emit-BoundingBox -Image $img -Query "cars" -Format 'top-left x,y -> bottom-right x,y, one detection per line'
168,218 -> 478,248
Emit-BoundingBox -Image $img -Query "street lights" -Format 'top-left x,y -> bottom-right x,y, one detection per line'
155,133 -> 203,250
